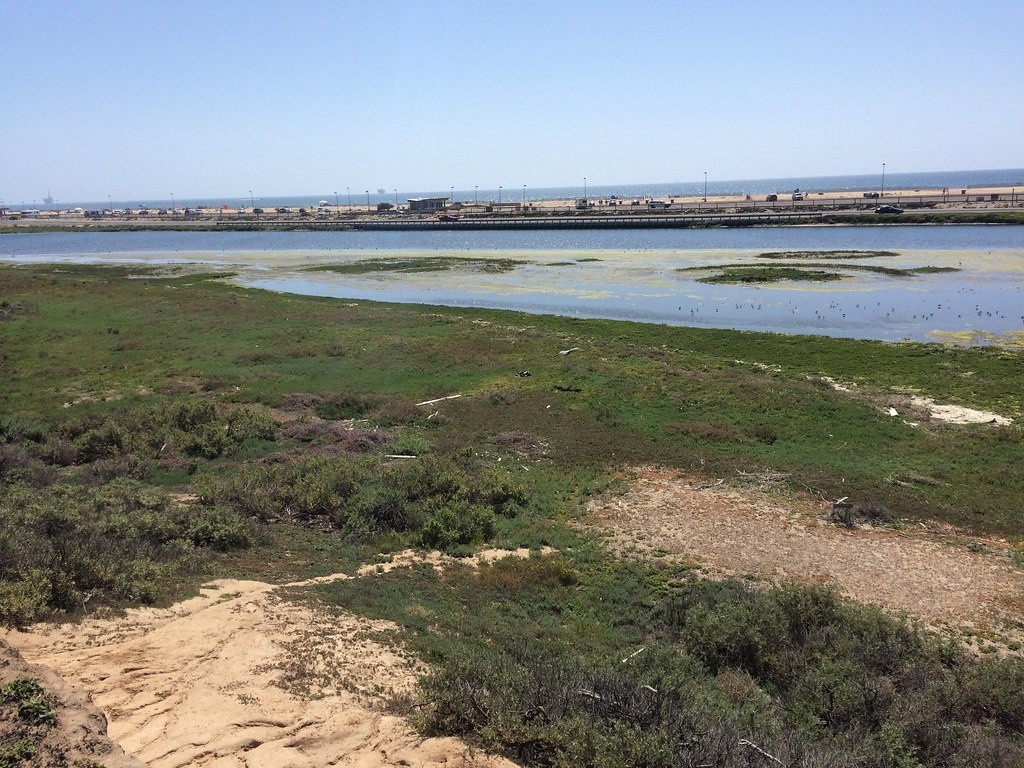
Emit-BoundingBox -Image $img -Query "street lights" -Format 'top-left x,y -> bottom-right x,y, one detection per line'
584,177 -> 587,200
880,162 -> 886,196
523,185 -> 526,206
499,186 -> 502,202
346,186 -> 351,210
451,186 -> 454,204
703,171 -> 708,201
475,185 -> 478,205
108,194 -> 113,211
394,189 -> 398,205
365,190 -> 370,210
170,192 -> 176,210
334,191 -> 339,209
249,190 -> 254,207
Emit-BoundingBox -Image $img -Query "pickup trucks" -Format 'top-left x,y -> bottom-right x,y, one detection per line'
381,209 -> 405,215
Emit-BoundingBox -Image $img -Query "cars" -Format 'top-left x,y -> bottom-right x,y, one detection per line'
158,209 -> 168,215
576,199 -> 640,209
867,192 -> 879,199
875,206 -> 905,215
238,208 -> 246,214
113,209 -> 148,218
174,208 -> 203,217
765,193 -> 803,202
253,207 -> 331,218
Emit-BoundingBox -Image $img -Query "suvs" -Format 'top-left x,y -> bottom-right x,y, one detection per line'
648,200 -> 672,209
